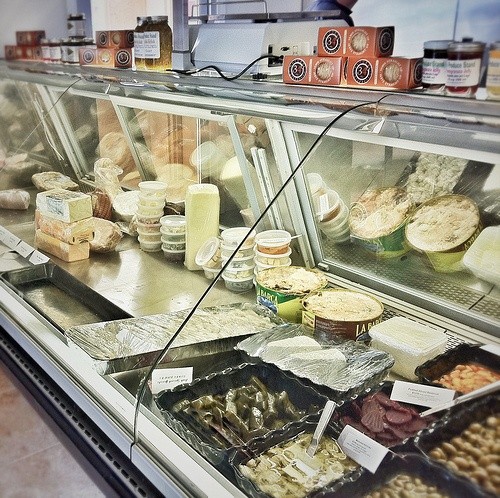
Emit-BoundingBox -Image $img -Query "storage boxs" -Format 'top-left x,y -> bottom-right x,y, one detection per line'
282,26 -> 424,89
80,30 -> 135,68
5,30 -> 46,59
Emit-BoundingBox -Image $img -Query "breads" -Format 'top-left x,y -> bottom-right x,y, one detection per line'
73,103 -> 270,205
0,172 -> 122,263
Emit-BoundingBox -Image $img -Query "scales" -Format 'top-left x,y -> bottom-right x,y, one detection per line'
186,1 -> 355,79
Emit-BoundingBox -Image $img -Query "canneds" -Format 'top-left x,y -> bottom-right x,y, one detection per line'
133,15 -> 173,71
420,40 -> 500,102
6,13 -> 96,63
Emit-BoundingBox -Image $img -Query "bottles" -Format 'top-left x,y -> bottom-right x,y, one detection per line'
66,12 -> 87,37
40,38 -> 96,67
420,41 -> 500,100
133,15 -> 172,70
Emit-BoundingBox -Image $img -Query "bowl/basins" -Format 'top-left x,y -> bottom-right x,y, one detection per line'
366,316 -> 447,380
307,174 -> 351,245
154,321 -> 500,498
459,226 -> 499,290
113,181 -> 293,291
